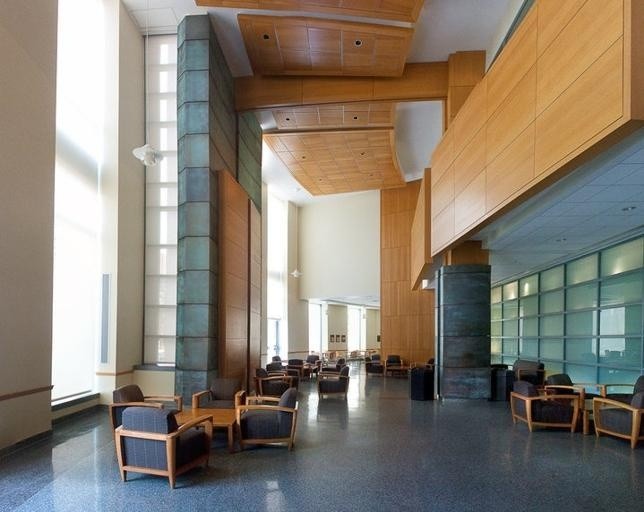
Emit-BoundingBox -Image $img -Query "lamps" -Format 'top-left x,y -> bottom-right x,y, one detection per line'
131,145 -> 163,169
290,270 -> 304,279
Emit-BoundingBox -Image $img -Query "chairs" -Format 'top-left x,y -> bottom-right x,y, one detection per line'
272,356 -> 287,368
545,373 -> 606,408
286,359 -> 310,381
364,357 -> 384,376
306,355 -> 320,372
592,375 -> 644,450
114,406 -> 212,489
318,358 -> 345,379
512,360 -> 545,384
108,383 -> 183,437
253,368 -> 299,396
598,377 -> 644,409
510,380 -> 579,433
236,388 -> 298,452
384,355 -> 403,376
316,366 -> 350,400
191,374 -> 246,409
